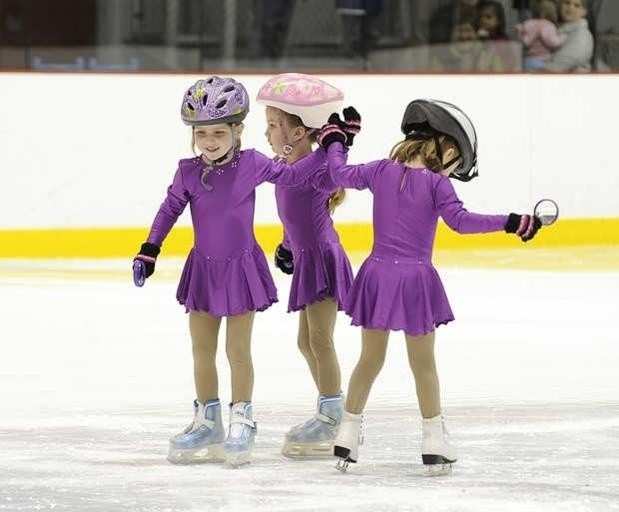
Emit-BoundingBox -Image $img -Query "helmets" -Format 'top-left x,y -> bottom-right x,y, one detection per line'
180,75 -> 250,126
400,97 -> 479,183
255,72 -> 345,131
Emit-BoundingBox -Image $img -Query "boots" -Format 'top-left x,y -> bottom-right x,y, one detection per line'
283,392 -> 346,443
332,407 -> 365,464
222,400 -> 258,454
419,412 -> 459,465
168,397 -> 226,450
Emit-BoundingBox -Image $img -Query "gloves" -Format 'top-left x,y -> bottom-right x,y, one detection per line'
132,242 -> 161,279
503,212 -> 543,243
315,105 -> 362,154
274,243 -> 294,274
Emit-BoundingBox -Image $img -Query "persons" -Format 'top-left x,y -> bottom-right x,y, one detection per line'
129,75 -> 362,454
314,96 -> 542,465
253,72 -> 363,444
428,0 -> 595,72
